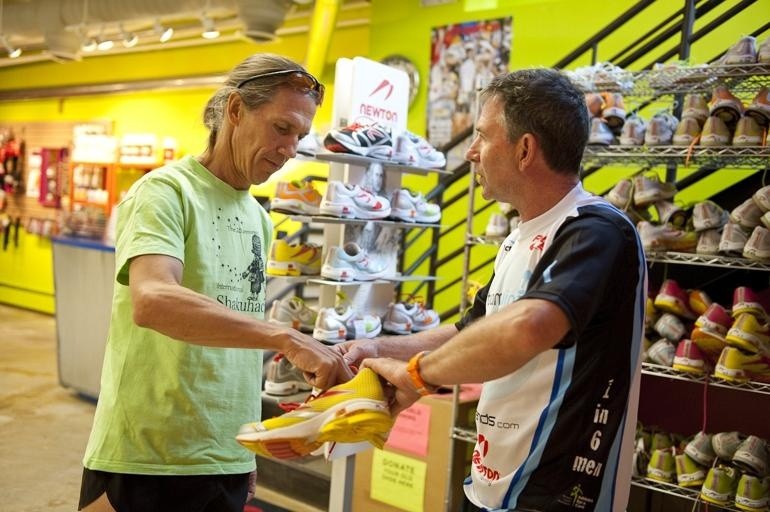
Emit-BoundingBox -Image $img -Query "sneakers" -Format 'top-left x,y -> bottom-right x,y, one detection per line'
270,296 -> 442,344
721,35 -> 770,64
607,174 -> 769,260
643,279 -> 769,383
264,238 -> 394,282
236,349 -> 393,465
634,425 -> 768,512
270,181 -> 442,223
587,89 -> 770,145
322,121 -> 446,169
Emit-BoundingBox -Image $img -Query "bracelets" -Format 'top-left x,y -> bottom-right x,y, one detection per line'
408,350 -> 446,396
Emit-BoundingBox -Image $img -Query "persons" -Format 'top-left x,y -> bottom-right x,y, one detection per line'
0,157 -> 24,252
327,65 -> 649,510
77,50 -> 354,512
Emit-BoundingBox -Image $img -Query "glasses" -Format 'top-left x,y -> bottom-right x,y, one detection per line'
239,69 -> 327,105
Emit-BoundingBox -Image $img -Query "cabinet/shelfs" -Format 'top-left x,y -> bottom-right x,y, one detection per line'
252,62 -> 770,512
67,158 -> 163,222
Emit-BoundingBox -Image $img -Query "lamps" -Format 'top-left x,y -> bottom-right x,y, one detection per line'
74,14 -> 219,51
1,33 -> 24,59
